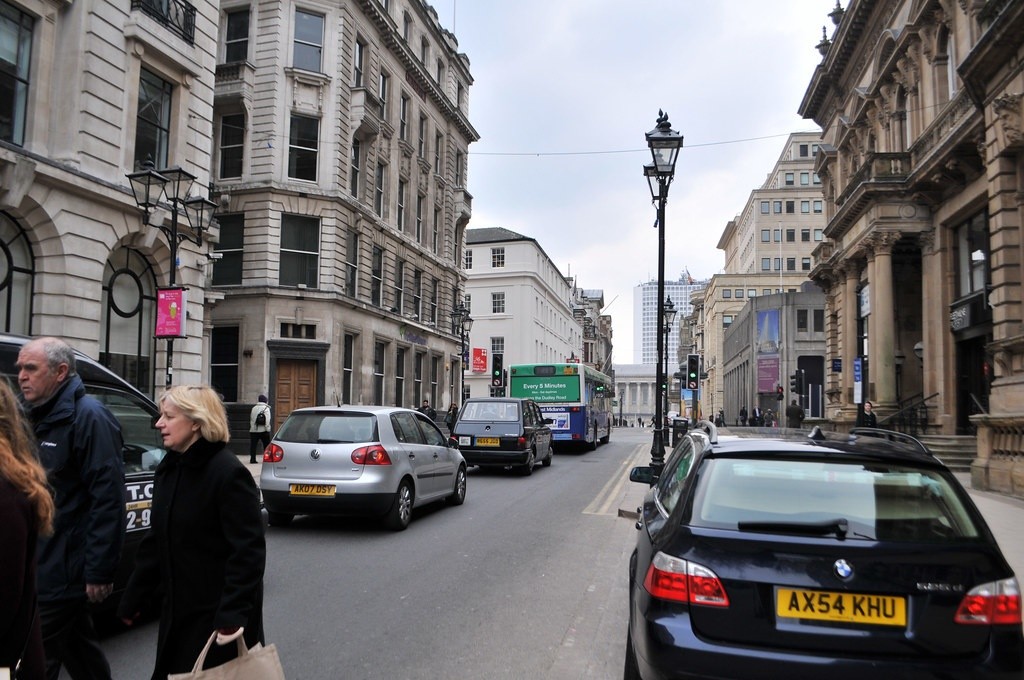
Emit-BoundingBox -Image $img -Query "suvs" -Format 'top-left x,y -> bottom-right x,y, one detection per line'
624,420 -> 1024,680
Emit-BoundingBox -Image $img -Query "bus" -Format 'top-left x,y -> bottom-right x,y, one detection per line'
510,364 -> 612,451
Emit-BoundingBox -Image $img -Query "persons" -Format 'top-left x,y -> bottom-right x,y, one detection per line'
0,380 -> 56,680
250,394 -> 272,463
649,415 -> 656,427
740,406 -> 748,426
443,403 -> 459,433
15,337 -> 126,680
752,405 -> 774,427
719,408 -> 727,427
854,402 -> 877,427
638,417 -> 644,427
417,400 -> 437,421
118,383 -> 266,680
787,399 -> 805,428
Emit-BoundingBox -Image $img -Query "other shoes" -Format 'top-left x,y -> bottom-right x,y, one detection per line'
249,459 -> 257,464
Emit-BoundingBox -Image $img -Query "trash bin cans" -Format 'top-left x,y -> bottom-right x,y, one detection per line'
672,418 -> 688,448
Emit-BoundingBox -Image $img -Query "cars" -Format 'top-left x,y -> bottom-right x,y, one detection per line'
0,335 -> 166,601
453,397 -> 553,476
667,411 -> 678,425
259,404 -> 469,530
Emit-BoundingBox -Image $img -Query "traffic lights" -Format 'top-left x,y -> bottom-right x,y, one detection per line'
687,354 -> 700,390
662,373 -> 667,391
595,382 -> 603,393
681,374 -> 686,389
492,354 -> 503,386
778,386 -> 783,394
790,370 -> 802,394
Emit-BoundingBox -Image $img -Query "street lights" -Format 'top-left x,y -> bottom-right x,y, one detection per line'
663,295 -> 677,448
450,301 -> 473,407
126,161 -> 217,389
641,108 -> 683,487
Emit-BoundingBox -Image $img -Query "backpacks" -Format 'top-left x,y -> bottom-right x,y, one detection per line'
255,405 -> 269,430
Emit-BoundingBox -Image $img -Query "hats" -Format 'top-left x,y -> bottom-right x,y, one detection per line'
258,395 -> 266,402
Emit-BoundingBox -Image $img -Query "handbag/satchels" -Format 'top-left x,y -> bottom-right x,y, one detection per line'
443,413 -> 453,423
169,625 -> 283,680
740,415 -> 745,421
